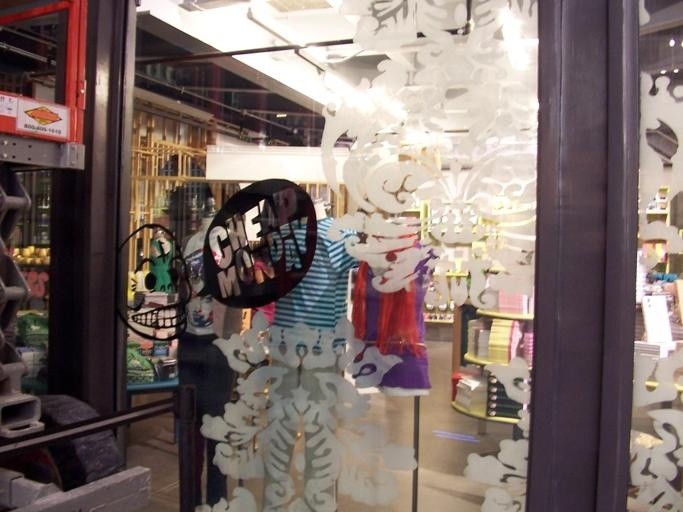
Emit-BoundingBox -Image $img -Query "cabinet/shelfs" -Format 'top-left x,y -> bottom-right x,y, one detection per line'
425,228 -> 471,322
452,298 -> 529,421
29,142 -> 215,315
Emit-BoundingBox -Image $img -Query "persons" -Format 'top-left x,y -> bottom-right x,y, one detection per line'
348,215 -> 443,399
173,217 -> 246,511
149,231 -> 173,292
259,197 -> 360,511
